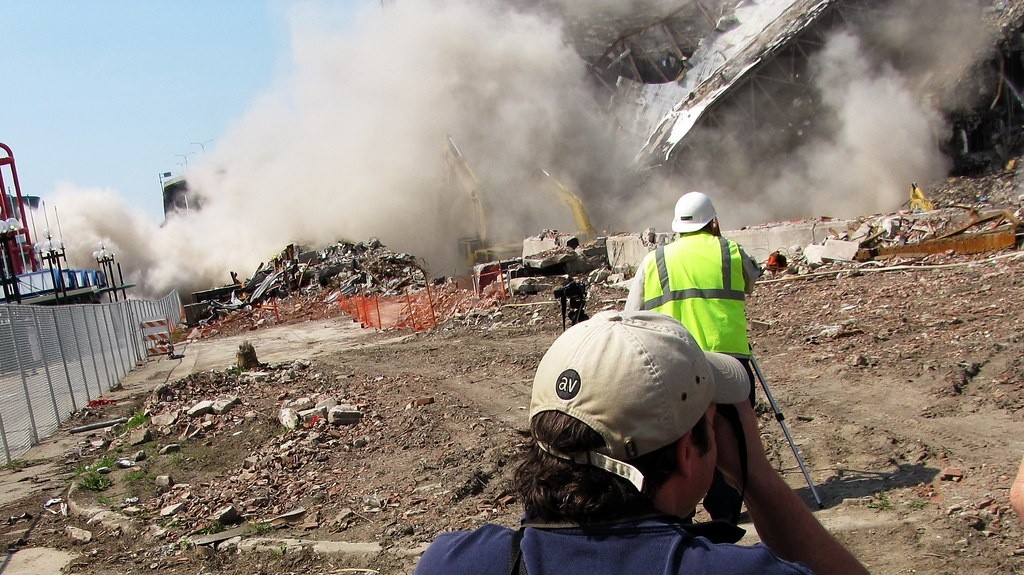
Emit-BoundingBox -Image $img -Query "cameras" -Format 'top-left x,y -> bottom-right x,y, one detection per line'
554,280 -> 587,310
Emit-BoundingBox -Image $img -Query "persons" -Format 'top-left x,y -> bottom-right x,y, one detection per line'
414,310 -> 872,575
625,189 -> 763,527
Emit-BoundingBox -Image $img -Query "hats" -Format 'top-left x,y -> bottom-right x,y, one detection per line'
525,309 -> 751,492
671,190 -> 721,233
